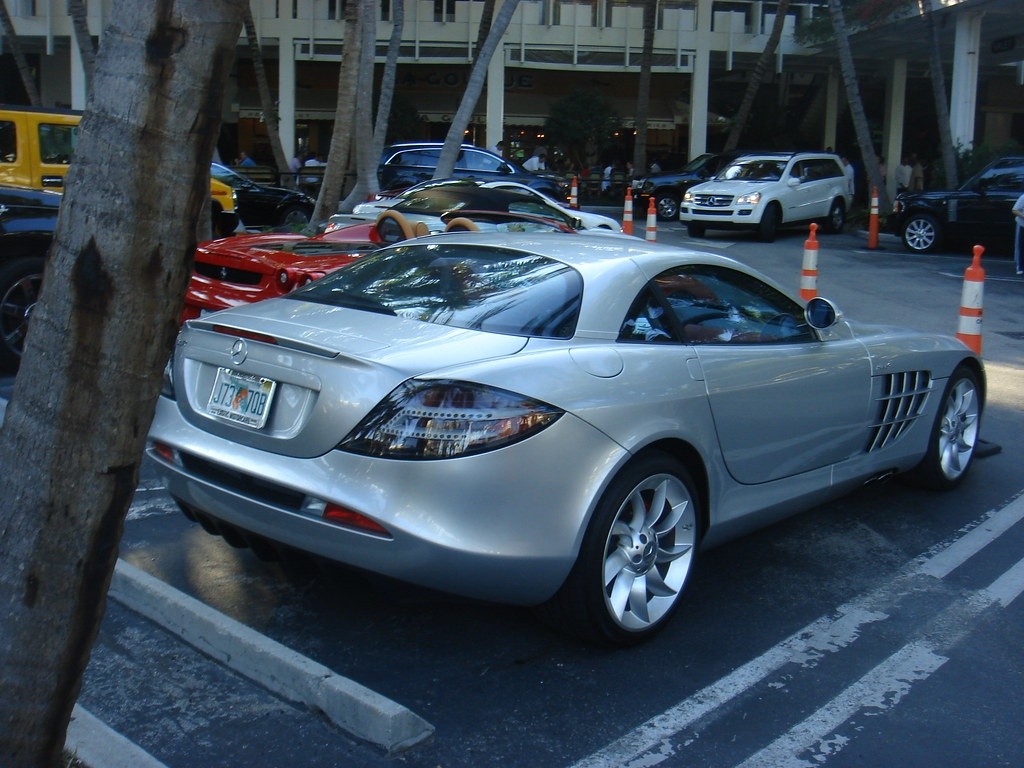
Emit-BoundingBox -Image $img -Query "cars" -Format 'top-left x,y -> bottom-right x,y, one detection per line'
376,141 -> 570,207
630,153 -> 740,222
325,177 -> 622,234
178,224 -> 385,323
887,155 -> 1024,255
0,185 -> 64,369
144,230 -> 987,648
210,162 -> 315,232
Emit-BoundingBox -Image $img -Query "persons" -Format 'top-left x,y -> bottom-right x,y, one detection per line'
1012,194 -> 1024,274
496,141 -> 515,163
522,141 -> 574,172
598,159 -> 613,179
647,159 -> 661,173
581,163 -> 591,179
657,269 -> 778,343
842,156 -> 854,201
876,153 -> 925,191
289,150 -> 327,183
626,160 -> 634,177
238,151 -> 257,166
827,147 -> 832,153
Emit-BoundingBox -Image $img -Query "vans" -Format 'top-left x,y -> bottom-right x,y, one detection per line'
0,109 -> 240,237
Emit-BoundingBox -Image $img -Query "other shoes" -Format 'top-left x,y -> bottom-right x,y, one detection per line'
1017,271 -> 1023,274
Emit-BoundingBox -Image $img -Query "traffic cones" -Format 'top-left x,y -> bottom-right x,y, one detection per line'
570,176 -> 577,209
623,187 -> 633,235
955,244 -> 1003,458
646,197 -> 657,242
799,223 -> 819,301
862,186 -> 885,250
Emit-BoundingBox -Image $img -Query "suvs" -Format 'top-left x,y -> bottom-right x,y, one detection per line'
679,149 -> 851,242
